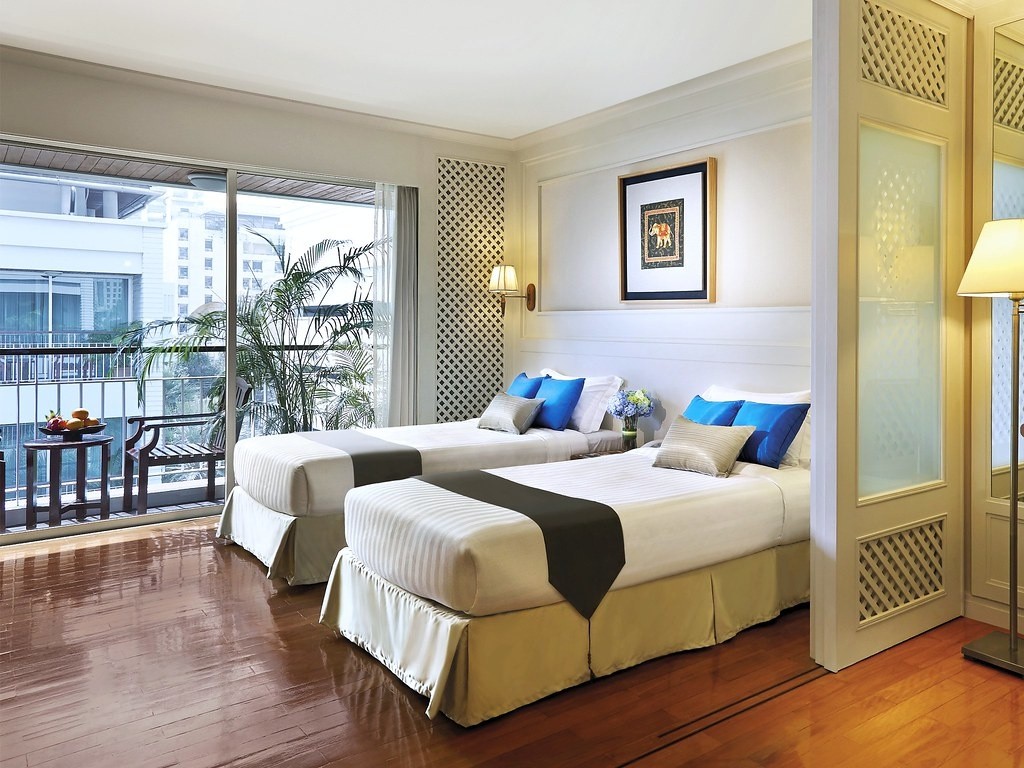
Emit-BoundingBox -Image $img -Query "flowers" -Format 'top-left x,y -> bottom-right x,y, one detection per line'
606,388 -> 656,451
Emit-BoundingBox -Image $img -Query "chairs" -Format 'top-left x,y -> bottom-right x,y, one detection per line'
123,376 -> 253,516
0,451 -> 6,533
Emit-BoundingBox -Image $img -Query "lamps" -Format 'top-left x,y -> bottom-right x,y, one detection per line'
187,173 -> 226,191
955,218 -> 1024,676
488,265 -> 535,317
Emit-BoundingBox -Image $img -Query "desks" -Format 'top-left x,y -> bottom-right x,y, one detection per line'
23,435 -> 114,530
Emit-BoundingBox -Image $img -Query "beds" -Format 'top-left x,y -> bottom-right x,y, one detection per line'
324,451 -> 810,728
214,418 -> 622,587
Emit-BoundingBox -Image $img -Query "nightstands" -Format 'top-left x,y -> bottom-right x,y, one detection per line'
568,450 -> 628,467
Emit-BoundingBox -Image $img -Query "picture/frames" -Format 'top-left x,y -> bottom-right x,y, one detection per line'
617,156 -> 717,303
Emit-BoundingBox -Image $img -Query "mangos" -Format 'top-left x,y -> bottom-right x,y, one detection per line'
72,409 -> 88,419
66,418 -> 84,429
84,418 -> 99,426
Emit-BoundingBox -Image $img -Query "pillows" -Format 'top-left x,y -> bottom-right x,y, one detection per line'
506,373 -> 552,399
535,368 -> 625,433
652,414 -> 756,478
701,385 -> 811,467
732,401 -> 811,469
534,374 -> 586,431
477,390 -> 547,435
682,395 -> 745,426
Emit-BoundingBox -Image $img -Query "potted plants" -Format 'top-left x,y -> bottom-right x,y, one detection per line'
89,320 -> 141,381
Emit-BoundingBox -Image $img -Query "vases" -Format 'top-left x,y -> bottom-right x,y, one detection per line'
620,413 -> 639,453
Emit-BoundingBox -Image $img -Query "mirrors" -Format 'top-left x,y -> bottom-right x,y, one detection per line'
989,19 -> 1024,503
